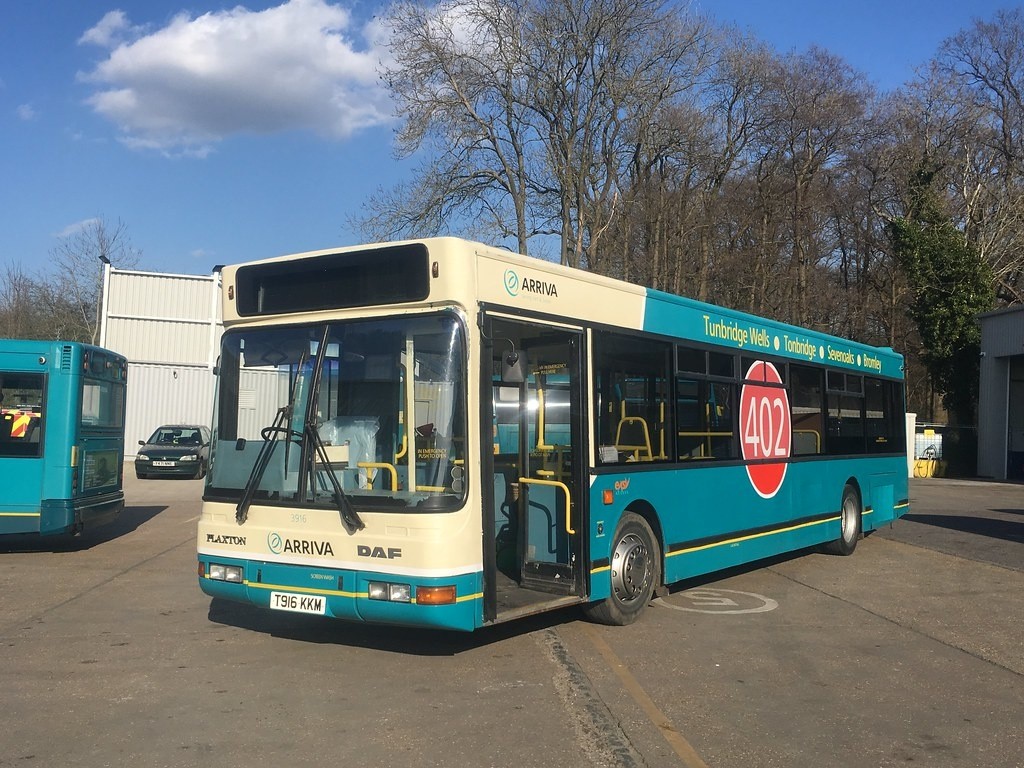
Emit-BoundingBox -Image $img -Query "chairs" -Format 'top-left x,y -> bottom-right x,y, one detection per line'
0,413 -> 15,446
164,433 -> 174,442
23,416 -> 40,442
317,417 -> 378,487
191,432 -> 200,444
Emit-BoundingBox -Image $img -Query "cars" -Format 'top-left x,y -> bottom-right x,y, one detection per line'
134,424 -> 211,479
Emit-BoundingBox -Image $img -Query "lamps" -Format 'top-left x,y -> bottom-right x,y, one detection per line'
979,352 -> 986,358
99,255 -> 111,267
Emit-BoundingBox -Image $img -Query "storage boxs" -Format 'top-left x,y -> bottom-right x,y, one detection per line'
916,434 -> 942,460
914,460 -> 945,477
924,430 -> 935,436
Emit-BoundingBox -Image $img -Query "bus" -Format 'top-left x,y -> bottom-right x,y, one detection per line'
195,237 -> 911,634
0,338 -> 129,538
